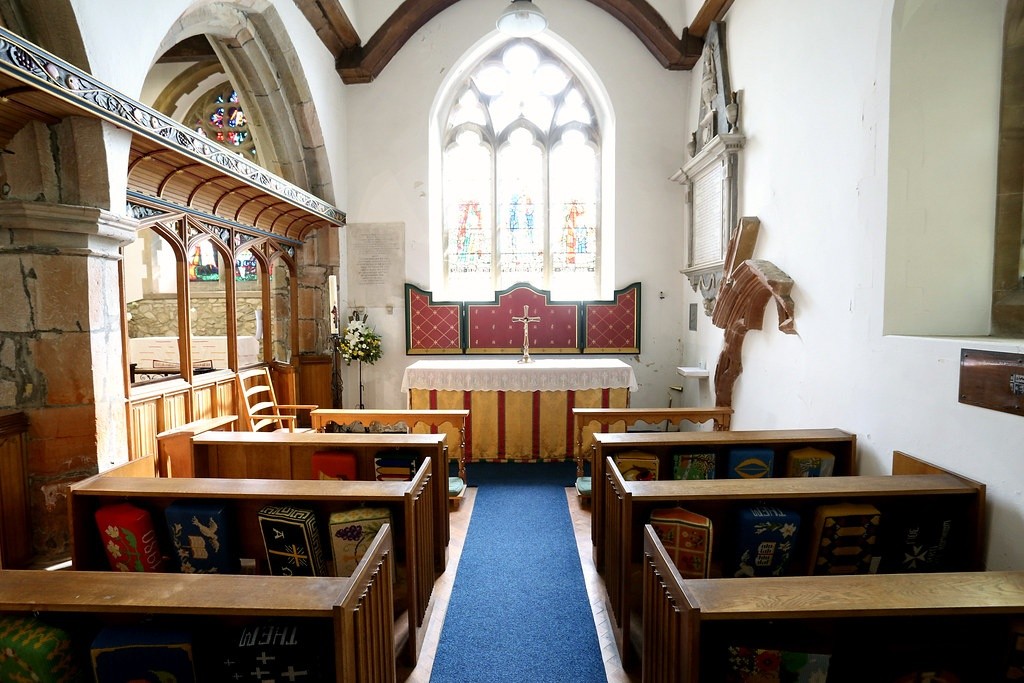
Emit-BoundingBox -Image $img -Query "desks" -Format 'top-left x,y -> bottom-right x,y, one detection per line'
400,359 -> 638,467
572,407 -> 1024,683
0,409 -> 471,683
0,408 -> 35,570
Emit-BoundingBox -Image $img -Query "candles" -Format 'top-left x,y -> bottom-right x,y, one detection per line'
328,275 -> 339,334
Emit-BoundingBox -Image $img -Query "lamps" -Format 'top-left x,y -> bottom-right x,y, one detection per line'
497,0 -> 548,37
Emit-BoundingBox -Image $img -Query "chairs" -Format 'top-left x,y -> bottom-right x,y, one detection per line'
235,366 -> 318,434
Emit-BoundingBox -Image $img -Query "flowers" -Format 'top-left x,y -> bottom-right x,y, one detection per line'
334,300 -> 385,367
